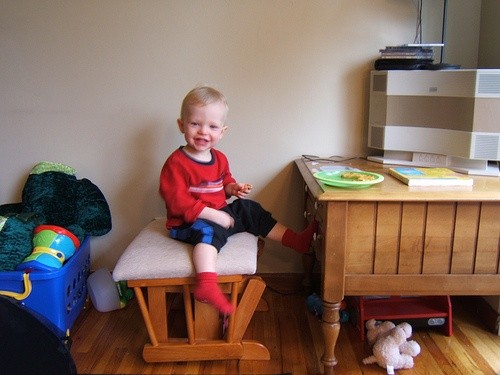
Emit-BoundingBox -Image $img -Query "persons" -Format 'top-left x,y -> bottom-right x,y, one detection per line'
159,86 -> 315,312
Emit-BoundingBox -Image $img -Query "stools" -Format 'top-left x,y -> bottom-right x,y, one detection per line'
113,216 -> 271,363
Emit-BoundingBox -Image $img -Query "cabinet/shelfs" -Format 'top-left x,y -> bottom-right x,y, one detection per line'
295,157 -> 500,375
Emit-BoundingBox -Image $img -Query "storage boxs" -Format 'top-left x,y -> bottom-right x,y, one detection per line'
0,232 -> 93,336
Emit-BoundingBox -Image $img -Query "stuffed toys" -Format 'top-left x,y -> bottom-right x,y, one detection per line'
363,319 -> 420,369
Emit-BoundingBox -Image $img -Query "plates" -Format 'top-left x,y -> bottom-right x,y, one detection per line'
313,170 -> 384,188
319,166 -> 360,171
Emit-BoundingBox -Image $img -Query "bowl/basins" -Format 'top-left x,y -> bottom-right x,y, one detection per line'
87,267 -> 129,312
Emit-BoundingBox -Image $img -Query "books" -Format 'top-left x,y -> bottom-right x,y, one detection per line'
387,167 -> 473,186
